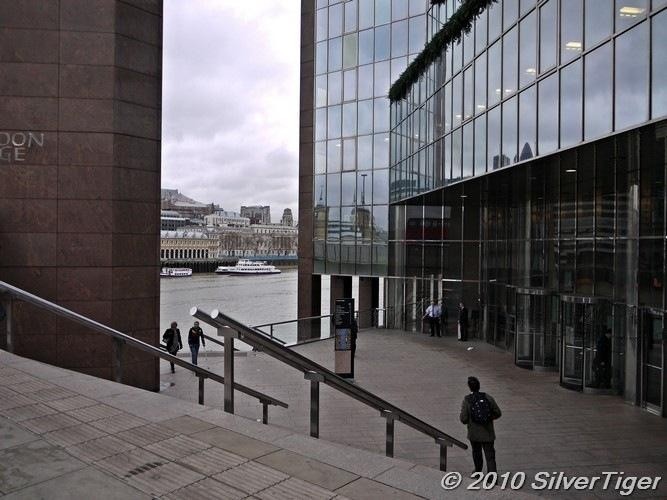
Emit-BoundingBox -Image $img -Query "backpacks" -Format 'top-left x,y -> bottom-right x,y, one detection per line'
469,392 -> 491,425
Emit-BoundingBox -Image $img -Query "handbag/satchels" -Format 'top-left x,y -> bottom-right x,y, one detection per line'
162,338 -> 169,343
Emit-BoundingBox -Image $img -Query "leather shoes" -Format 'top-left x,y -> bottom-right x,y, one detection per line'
429,334 -> 434,337
437,335 -> 442,337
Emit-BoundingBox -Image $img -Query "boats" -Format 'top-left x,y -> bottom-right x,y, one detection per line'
160,267 -> 192,276
215,258 -> 282,276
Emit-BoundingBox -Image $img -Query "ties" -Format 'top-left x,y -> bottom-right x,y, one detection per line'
432,305 -> 434,317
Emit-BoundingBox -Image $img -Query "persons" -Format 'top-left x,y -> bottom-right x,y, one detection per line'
423,300 -> 441,337
460,377 -> 501,484
162,321 -> 205,373
598,328 -> 612,389
456,301 -> 468,341
